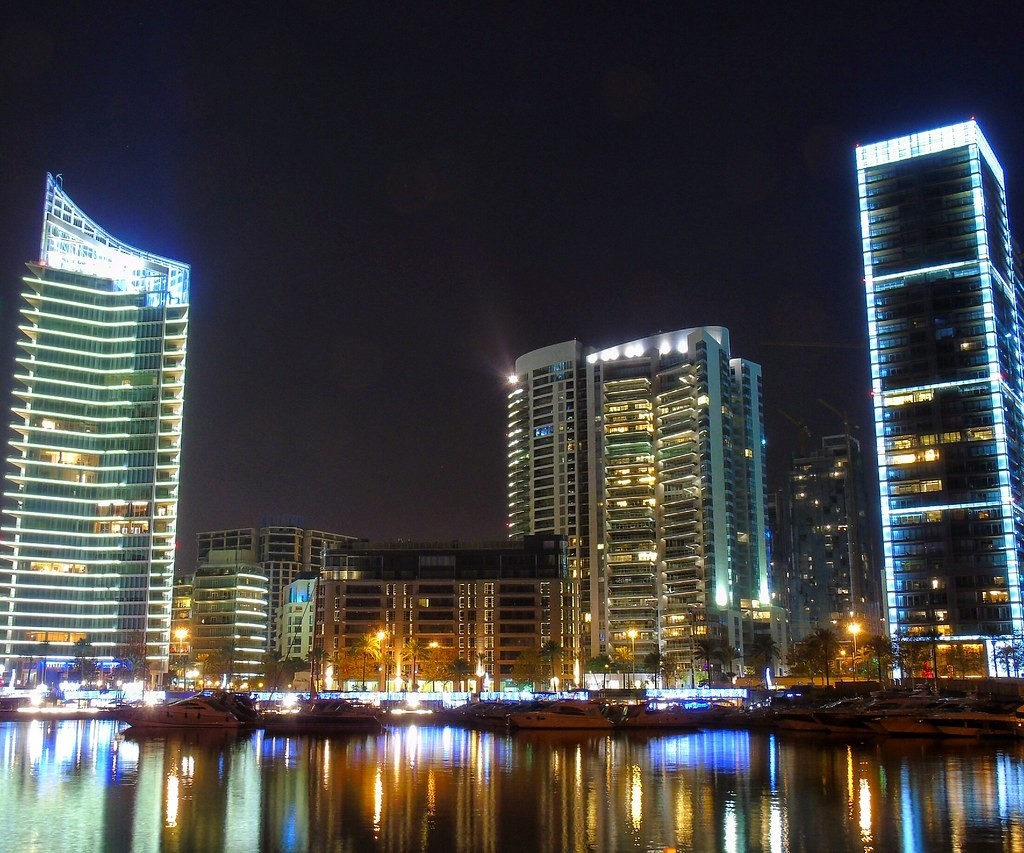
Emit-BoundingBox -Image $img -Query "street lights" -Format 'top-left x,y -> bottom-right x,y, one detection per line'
626,628 -> 639,689
850,624 -> 861,680
929,576 -> 939,678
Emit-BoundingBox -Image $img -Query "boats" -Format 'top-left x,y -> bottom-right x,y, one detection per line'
599,702 -> 705,730
920,705 -> 1014,735
1008,704 -> 1024,737
862,712 -> 941,735
432,700 -> 555,727
509,700 -> 613,726
109,689 -> 258,727
692,697 -> 800,728
765,709 -> 819,730
809,710 -> 874,733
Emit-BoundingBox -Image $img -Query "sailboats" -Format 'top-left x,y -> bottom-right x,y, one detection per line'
260,569 -> 394,733
